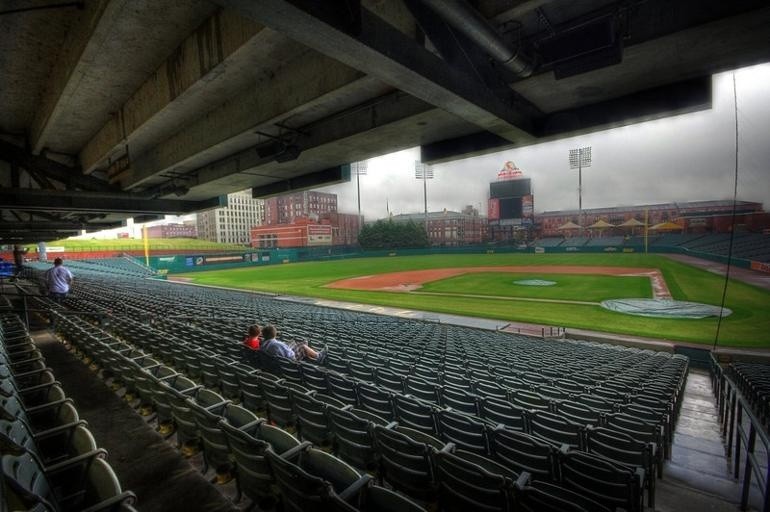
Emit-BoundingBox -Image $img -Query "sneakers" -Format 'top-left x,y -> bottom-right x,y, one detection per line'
316,344 -> 328,364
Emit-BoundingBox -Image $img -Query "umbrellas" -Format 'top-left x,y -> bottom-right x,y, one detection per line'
648,222 -> 683,232
586,220 -> 616,239
616,218 -> 653,236
558,221 -> 582,238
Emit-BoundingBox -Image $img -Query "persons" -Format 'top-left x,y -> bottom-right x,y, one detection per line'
45,257 -> 72,330
13,245 -> 29,277
243,324 -> 261,349
261,325 -> 328,364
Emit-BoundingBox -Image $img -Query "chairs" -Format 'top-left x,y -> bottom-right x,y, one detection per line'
1,315 -> 690,512
1,258 -> 167,315
710,351 -> 770,512
167,280 -> 383,315
